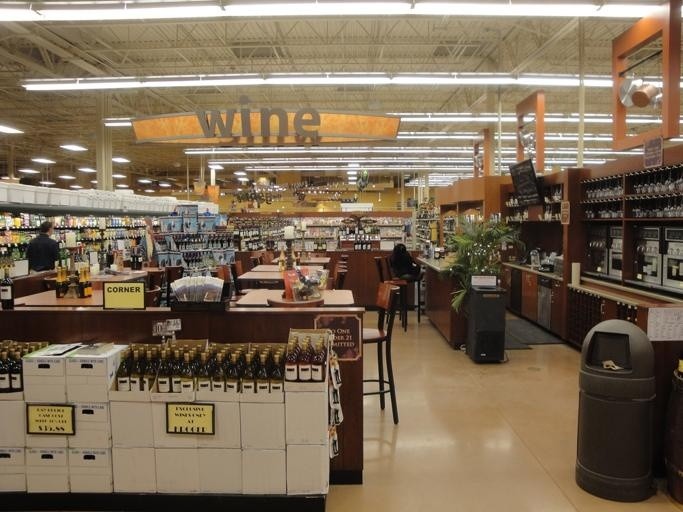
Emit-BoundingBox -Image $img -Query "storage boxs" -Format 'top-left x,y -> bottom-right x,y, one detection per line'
0,182 -> 219,214
0,344 -> 331,496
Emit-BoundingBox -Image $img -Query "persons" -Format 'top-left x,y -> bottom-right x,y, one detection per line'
391,242 -> 417,293
26,221 -> 61,275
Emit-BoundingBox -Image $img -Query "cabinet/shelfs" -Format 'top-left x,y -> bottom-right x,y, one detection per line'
505,175 -> 564,274
624,164 -> 683,295
580,168 -> 623,283
0,211 -> 483,286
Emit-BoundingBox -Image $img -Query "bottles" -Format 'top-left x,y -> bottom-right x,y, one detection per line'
285,335 -> 326,383
428,241 -> 434,257
130,247 -> 142,270
0,338 -> 51,392
177,252 -> 213,269
157,233 -> 233,250
278,244 -> 296,271
0,267 -> 15,310
354,236 -> 371,250
240,229 -> 263,251
54,265 -> 69,298
79,266 -> 93,298
313,234 -> 326,252
116,341 -> 283,395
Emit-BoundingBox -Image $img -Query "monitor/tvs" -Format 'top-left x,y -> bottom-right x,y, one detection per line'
509,159 -> 543,207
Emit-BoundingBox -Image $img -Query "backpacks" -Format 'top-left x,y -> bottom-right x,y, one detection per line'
391,243 -> 420,277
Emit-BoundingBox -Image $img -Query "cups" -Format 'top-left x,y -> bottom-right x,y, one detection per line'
433,251 -> 441,259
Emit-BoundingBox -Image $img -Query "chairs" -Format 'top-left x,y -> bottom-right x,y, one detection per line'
374,256 -> 408,329
389,244 -> 423,323
14,252 -> 356,307
363,282 -> 406,426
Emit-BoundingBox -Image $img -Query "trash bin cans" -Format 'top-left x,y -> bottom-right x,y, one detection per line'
574,318 -> 660,502
466,284 -> 508,364
663,369 -> 683,505
